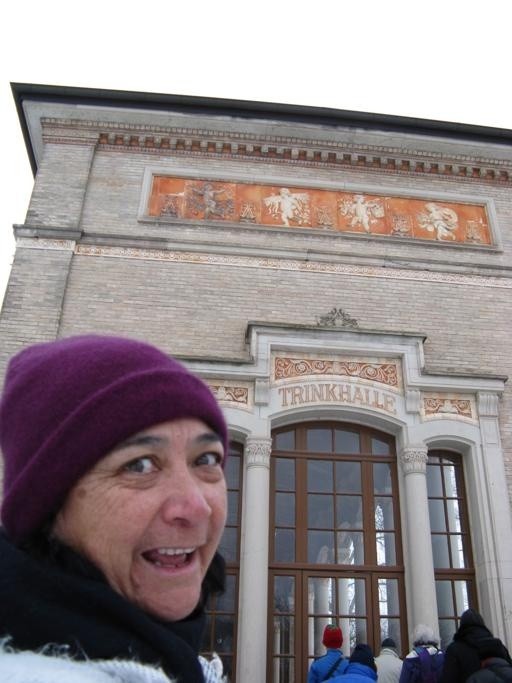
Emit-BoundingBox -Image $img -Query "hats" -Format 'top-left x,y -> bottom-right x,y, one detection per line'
322,623 -> 343,648
350,643 -> 374,663
380,637 -> 396,649
460,608 -> 484,626
1,334 -> 230,544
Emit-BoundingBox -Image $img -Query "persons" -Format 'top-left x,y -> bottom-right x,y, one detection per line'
308,624 -> 349,683
399,624 -> 445,683
373,638 -> 404,683
443,609 -> 512,683
322,644 -> 378,683
466,646 -> 512,683
0,333 -> 229,683
